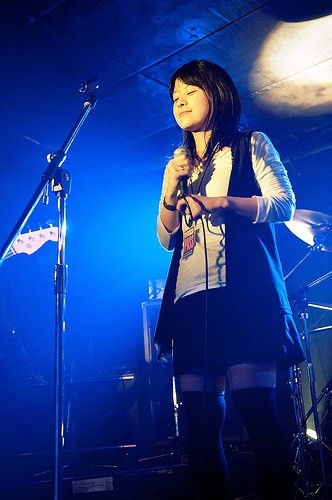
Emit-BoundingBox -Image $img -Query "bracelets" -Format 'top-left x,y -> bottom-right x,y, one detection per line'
162,195 -> 178,211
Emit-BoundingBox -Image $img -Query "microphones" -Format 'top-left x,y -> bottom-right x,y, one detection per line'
288,286 -> 310,300
174,147 -> 192,195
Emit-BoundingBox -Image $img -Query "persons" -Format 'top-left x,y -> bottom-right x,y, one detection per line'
151,57 -> 299,500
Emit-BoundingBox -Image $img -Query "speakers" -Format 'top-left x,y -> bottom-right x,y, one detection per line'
29,377 -> 147,448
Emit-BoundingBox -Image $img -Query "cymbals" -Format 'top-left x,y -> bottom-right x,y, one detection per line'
308,326 -> 332,333
284,210 -> 331,251
307,303 -> 331,310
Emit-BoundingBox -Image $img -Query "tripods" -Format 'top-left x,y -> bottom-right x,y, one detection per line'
288,316 -> 332,500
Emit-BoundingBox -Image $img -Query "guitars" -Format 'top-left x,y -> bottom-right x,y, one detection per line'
0,219 -> 58,260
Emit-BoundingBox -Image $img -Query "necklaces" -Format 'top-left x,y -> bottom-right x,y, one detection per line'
190,147 -> 209,174
